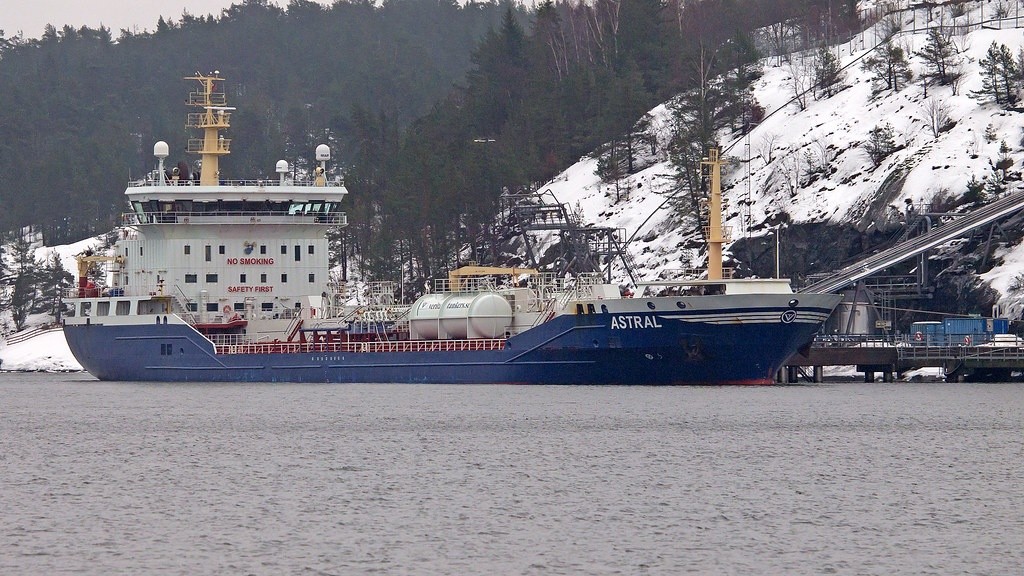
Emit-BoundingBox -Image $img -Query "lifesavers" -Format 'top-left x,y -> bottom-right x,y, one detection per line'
914,331 -> 923,341
223,304 -> 232,314
964,336 -> 970,345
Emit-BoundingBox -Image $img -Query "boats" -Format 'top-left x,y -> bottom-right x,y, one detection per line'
59,68 -> 847,387
